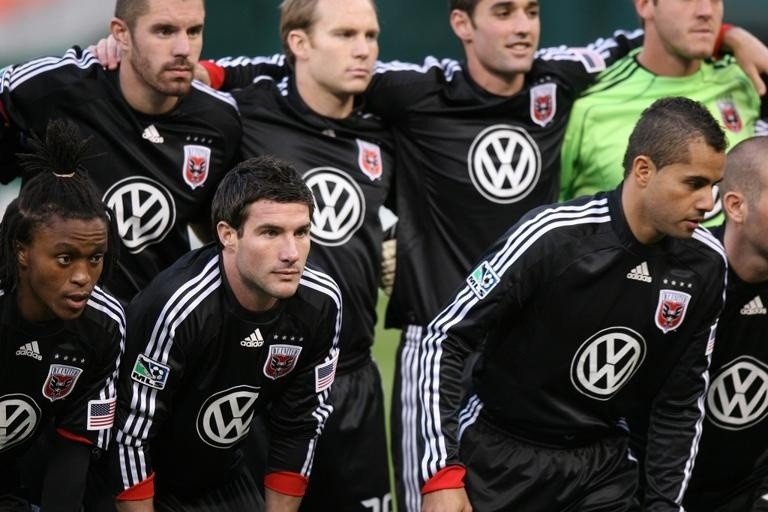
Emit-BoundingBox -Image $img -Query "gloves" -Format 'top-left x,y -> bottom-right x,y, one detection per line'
381,238 -> 398,297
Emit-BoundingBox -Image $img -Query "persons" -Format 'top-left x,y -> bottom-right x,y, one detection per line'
1,0 -> 243,307
391,96 -> 730,512
107,153 -> 345,511
681,134 -> 767,512
193,0 -> 768,512
1,118 -> 125,510
559,0 -> 768,246
89,0 -> 394,511
